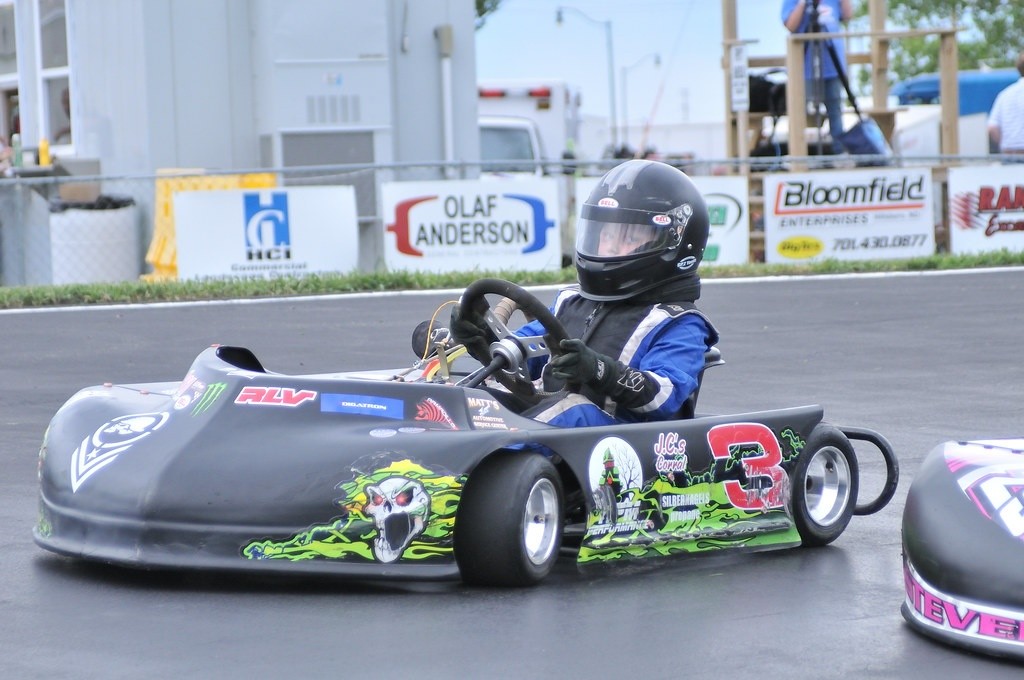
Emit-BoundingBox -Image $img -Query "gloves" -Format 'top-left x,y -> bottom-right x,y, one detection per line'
448,306 -> 488,348
551,338 -> 622,396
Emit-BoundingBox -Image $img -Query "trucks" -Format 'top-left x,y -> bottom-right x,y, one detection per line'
478,83 -> 580,172
886,70 -> 1021,118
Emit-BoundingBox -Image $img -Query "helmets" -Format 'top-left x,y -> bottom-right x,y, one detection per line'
575,159 -> 709,305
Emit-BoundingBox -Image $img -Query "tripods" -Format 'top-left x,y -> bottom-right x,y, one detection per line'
765,0 -> 862,155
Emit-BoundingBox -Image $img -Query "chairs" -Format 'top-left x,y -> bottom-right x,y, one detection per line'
676,346 -> 724,420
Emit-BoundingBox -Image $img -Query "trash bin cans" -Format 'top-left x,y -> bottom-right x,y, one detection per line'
50,193 -> 141,286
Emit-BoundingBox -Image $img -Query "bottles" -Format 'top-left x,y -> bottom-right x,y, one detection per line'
38,137 -> 49,166
12,134 -> 22,166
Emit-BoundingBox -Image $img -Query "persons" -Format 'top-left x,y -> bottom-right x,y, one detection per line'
985,51 -> 1024,159
777,0 -> 855,155
438,156 -> 721,425
